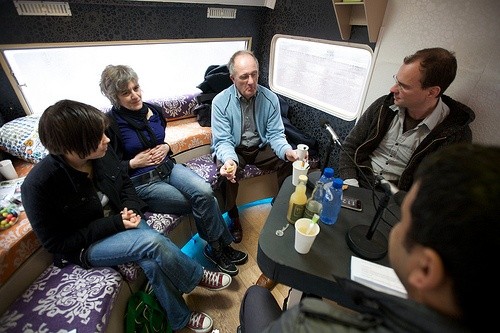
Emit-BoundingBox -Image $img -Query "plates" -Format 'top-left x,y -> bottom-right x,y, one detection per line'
0,207 -> 19,230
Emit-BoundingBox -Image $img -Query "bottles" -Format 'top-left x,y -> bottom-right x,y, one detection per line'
304,181 -> 324,225
287,174 -> 308,225
314,168 -> 343,225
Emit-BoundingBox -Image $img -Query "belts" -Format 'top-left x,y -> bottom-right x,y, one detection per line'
130,158 -> 173,186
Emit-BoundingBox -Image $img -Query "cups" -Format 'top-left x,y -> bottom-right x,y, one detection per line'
292,160 -> 310,186
294,218 -> 320,254
297,144 -> 309,160
0,159 -> 18,179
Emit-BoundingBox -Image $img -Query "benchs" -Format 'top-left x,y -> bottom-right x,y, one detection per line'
0,93 -> 313,333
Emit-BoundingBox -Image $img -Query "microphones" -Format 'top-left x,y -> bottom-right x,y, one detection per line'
377,176 -> 408,205
318,117 -> 339,143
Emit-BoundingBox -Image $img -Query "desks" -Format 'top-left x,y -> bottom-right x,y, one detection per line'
256,172 -> 406,314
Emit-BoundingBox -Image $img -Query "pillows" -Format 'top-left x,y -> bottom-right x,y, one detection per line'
0,111 -> 50,165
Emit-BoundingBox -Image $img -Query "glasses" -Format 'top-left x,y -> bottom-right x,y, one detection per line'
393,74 -> 435,97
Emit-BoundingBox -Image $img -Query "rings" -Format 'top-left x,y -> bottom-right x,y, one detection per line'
157,158 -> 160,160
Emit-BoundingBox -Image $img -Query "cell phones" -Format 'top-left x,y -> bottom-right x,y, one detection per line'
340,196 -> 363,212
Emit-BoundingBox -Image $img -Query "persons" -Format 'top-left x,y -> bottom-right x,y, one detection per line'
237,144 -> 500,333
339,47 -> 477,195
100,65 -> 248,275
20,100 -> 232,333
211,49 -> 309,242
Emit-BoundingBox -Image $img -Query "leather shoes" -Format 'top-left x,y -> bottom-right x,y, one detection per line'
237,325 -> 241,333
256,273 -> 275,290
229,218 -> 243,243
271,196 -> 277,205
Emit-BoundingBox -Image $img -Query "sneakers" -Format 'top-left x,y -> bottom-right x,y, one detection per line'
201,243 -> 239,276
180,311 -> 213,333
196,268 -> 232,291
222,246 -> 248,264
